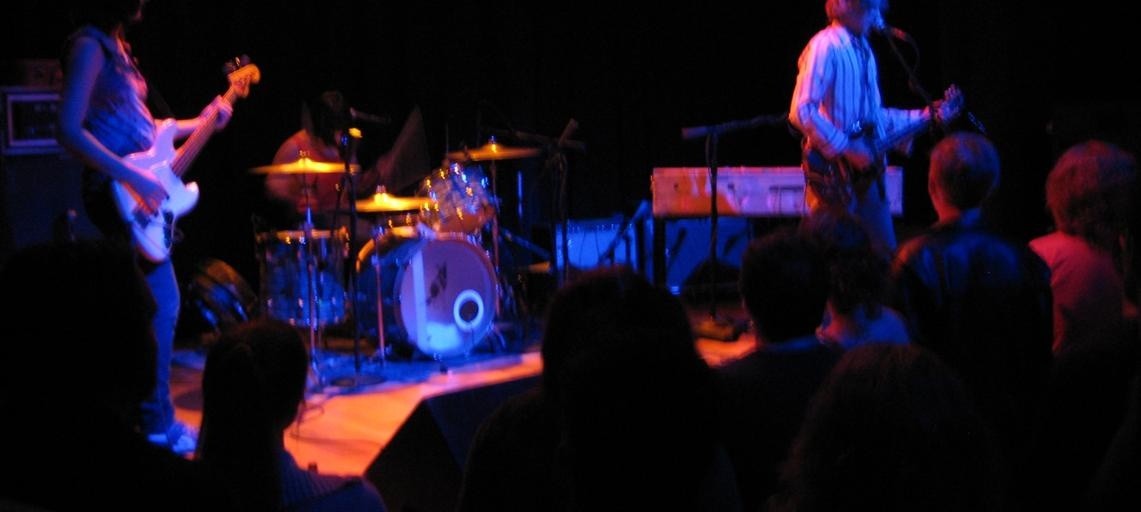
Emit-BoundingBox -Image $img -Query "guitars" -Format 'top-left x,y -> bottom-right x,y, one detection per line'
114,63 -> 259,264
802,88 -> 961,187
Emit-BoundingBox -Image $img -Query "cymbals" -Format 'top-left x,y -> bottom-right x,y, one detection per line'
354,190 -> 433,212
448,140 -> 537,161
258,154 -> 357,178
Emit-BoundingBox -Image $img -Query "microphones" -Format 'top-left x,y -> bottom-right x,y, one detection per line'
871,21 -> 911,43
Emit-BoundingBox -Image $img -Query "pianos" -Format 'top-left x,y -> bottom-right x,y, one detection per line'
652,162 -> 902,213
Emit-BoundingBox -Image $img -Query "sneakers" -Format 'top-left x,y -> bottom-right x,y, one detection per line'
146,425 -> 200,454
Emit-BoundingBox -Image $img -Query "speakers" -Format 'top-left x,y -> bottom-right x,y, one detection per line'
365,374 -> 543,512
556,217 -> 639,292
643,216 -> 755,299
3,154 -> 112,254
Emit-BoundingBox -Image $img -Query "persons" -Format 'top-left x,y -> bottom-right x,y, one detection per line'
452,133 -> 1141,512
50,1 -> 234,453
3,233 -> 386,508
263,88 -> 379,260
783,0 -> 962,252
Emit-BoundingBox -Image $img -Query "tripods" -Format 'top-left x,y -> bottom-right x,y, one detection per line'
353,226 -> 432,385
477,196 -> 524,350
298,220 -> 362,396
474,168 -> 550,262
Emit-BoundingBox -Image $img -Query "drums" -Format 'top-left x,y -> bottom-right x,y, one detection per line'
263,226 -> 347,331
354,224 -> 502,363
419,164 -> 496,235
